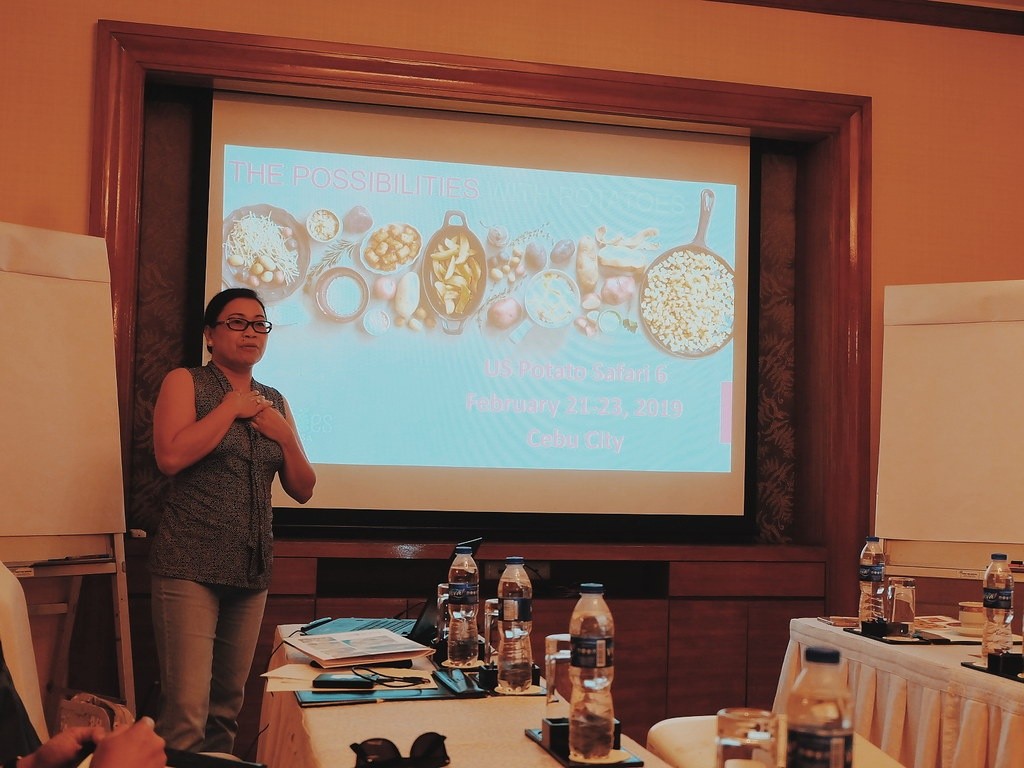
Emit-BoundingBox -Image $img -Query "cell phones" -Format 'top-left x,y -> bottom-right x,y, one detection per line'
818,617 -> 858,627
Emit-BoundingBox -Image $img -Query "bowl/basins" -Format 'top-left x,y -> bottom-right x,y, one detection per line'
315,266 -> 369,322
306,209 -> 343,242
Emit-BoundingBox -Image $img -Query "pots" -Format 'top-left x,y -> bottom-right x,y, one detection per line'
510,269 -> 581,343
640,188 -> 735,360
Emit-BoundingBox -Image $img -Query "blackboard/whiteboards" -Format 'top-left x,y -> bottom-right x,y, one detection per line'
877,279 -> 1023,582
0,221 -> 126,574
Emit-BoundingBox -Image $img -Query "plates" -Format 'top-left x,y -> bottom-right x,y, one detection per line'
945,622 -> 983,636
363,308 -> 391,335
422,210 -> 488,336
598,308 -> 622,334
360,222 -> 423,274
221,203 -> 311,302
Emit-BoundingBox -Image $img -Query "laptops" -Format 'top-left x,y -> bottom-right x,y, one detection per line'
307,537 -> 483,637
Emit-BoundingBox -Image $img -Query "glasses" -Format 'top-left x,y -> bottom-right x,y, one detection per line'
212,317 -> 273,333
350,732 -> 451,768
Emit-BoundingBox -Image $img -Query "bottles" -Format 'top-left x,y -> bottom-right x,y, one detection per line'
982,553 -> 1014,666
448,547 -> 479,666
786,647 -> 854,768
859,537 -> 885,630
497,557 -> 533,691
569,583 -> 614,760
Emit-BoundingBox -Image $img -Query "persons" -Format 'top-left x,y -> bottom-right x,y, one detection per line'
0,715 -> 166,768
148,288 -> 316,750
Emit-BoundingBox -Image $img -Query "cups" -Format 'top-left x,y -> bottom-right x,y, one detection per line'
484,599 -> 501,669
545,633 -> 572,717
959,602 -> 984,628
437,583 -> 449,650
886,577 -> 915,635
716,708 -> 778,768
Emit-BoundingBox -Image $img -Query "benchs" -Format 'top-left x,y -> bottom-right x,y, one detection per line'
646,713 -> 907,768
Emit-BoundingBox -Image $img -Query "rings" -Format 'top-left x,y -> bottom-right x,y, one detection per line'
257,398 -> 261,404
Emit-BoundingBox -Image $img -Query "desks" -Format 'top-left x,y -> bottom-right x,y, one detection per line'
255,624 -> 676,768
772,617 -> 1024,768
